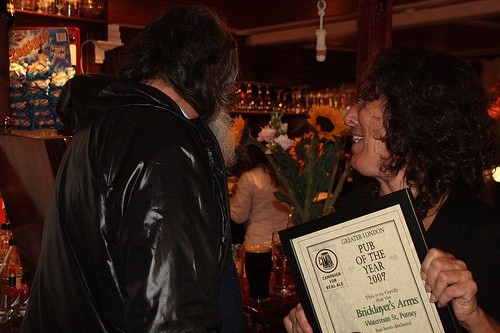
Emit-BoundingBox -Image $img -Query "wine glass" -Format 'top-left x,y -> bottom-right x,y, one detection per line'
236,80 -> 357,112
272,231 -> 296,296
56,0 -> 66,15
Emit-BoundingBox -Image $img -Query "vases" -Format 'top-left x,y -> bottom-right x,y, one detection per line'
268,231 -> 300,305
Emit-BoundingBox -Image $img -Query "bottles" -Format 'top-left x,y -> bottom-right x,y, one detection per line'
0,222 -> 30,324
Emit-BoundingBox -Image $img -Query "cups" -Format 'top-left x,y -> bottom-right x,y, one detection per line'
231,243 -> 244,280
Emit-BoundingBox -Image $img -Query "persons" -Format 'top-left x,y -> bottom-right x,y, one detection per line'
282,44 -> 499,333
230,144 -> 280,306
19,4 -> 243,333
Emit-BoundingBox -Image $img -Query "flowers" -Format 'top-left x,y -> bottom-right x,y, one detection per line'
227,112 -> 352,223
